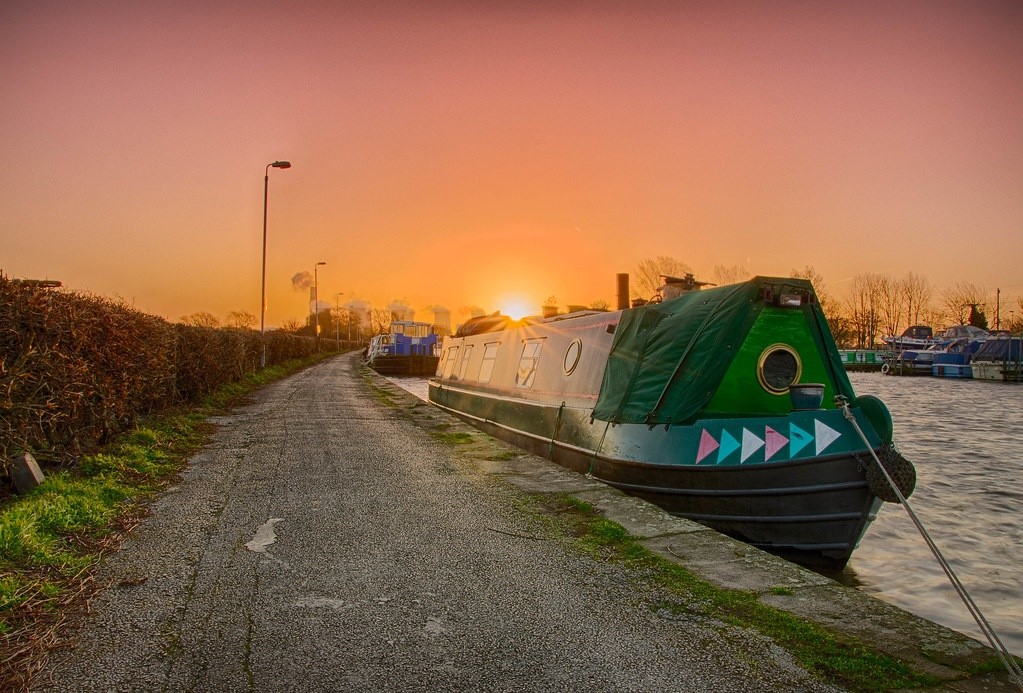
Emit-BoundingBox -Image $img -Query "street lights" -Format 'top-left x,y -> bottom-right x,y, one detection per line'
348,305 -> 354,349
336,293 -> 344,351
314,261 -> 327,335
257,160 -> 291,367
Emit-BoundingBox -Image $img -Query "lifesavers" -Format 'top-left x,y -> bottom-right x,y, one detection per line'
881,363 -> 890,373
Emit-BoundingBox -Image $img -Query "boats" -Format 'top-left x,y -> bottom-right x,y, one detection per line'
432,270 -> 918,582
883,325 -> 1023,384
367,323 -> 440,377
